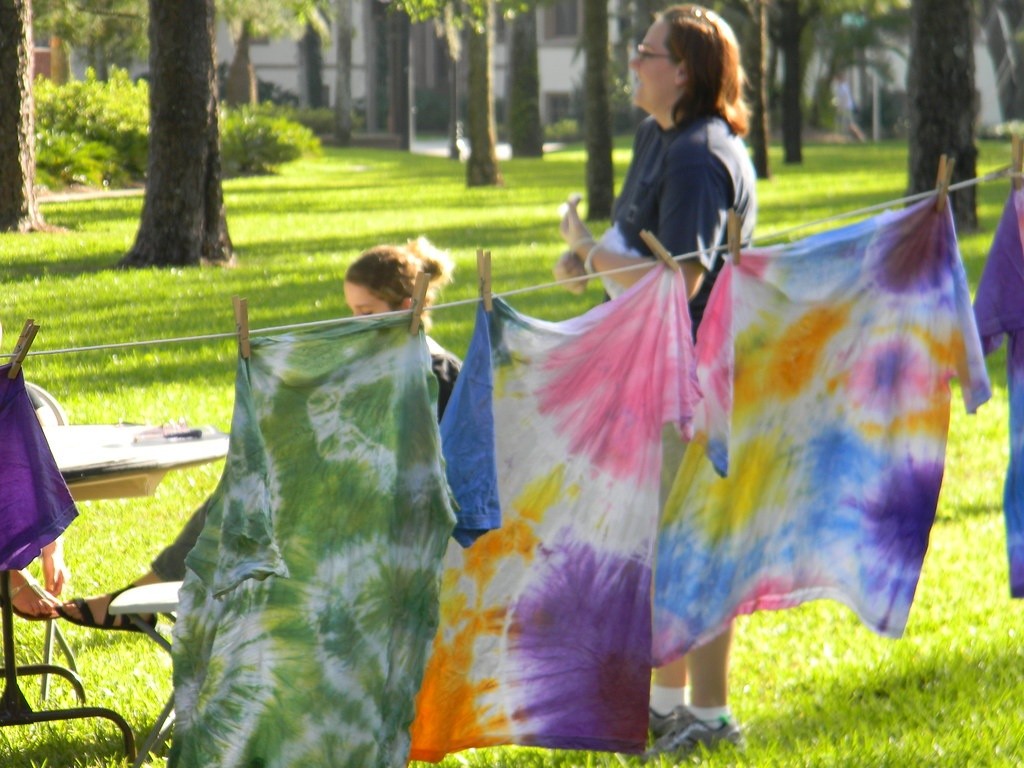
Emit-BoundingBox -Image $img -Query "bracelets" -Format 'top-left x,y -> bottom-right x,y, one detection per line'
571,238 -> 596,258
583,244 -> 598,276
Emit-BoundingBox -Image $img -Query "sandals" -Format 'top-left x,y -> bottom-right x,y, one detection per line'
11,580 -> 62,620
58,584 -> 158,632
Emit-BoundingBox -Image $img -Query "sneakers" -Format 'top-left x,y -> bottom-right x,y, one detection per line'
647,704 -> 743,760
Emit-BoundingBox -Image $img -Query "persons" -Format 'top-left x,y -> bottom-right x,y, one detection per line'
0,535 -> 69,621
553,3 -> 759,766
52,234 -> 462,633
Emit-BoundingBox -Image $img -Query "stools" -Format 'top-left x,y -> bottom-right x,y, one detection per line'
107,581 -> 204,767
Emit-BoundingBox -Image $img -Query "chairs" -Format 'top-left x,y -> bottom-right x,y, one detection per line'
24,380 -> 85,704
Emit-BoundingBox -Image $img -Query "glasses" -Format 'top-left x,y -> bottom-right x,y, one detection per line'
637,44 -> 671,64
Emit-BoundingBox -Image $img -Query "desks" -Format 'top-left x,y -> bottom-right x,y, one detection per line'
0,423 -> 233,763
